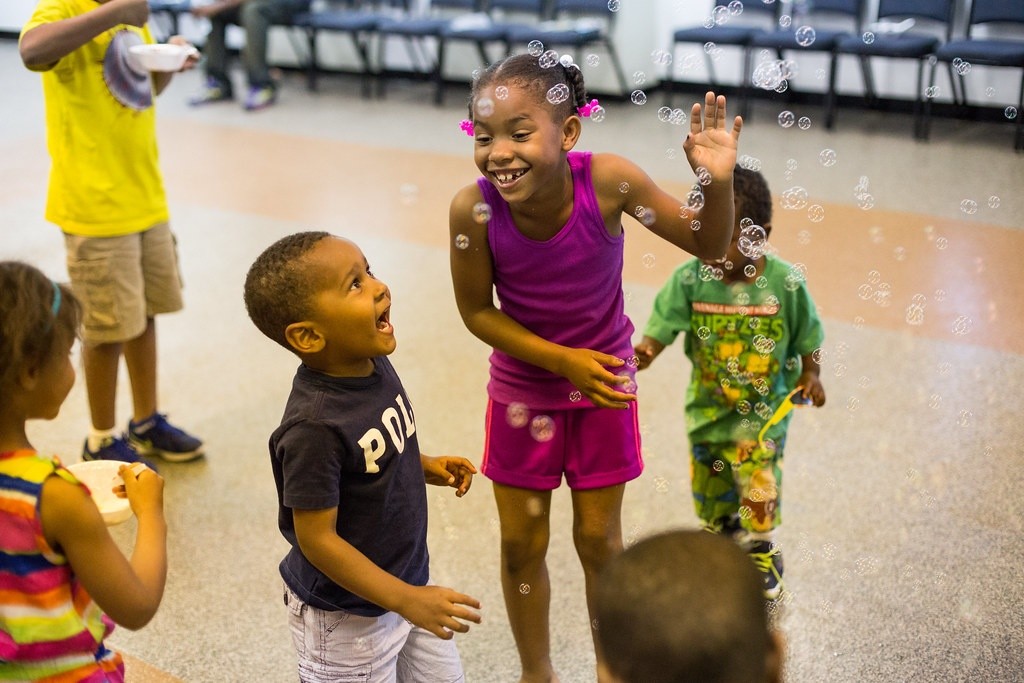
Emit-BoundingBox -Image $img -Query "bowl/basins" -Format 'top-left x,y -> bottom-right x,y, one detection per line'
128,43 -> 197,75
64,459 -> 137,525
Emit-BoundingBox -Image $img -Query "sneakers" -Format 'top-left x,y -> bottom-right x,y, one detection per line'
747,540 -> 784,600
698,515 -> 751,547
82,433 -> 156,473
129,412 -> 205,462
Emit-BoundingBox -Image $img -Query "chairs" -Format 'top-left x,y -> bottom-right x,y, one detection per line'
507,1 -> 630,101
150,1 -> 191,46
309,0 -> 375,95
376,0 -> 442,98
435,1 -> 510,101
735,1 -> 879,121
664,1 -> 795,117
826,0 -> 960,134
923,0 -> 1024,151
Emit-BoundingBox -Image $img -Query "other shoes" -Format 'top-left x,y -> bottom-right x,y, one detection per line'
187,78 -> 232,103
246,84 -> 278,109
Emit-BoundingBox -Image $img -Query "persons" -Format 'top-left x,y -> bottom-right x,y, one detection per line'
633,166 -> 827,596
188,2 -> 313,112
584,526 -> 787,683
244,228 -> 480,683
448,56 -> 745,683
20,0 -> 208,463
2,259 -> 179,683
